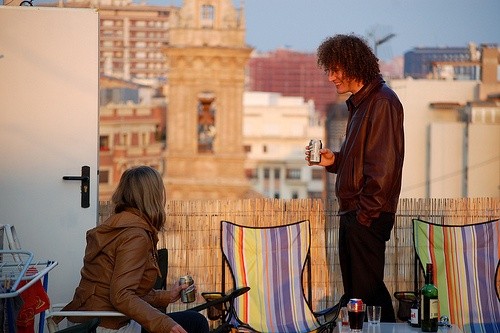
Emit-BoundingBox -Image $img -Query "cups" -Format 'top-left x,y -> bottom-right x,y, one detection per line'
348,311 -> 365,331
367,306 -> 381,326
362,304 -> 366,318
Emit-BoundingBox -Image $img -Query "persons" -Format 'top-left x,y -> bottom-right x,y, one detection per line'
304,33 -> 405,329
49,167 -> 190,333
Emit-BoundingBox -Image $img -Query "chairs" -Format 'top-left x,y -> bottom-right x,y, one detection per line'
220,219 -> 337,333
5,223 -> 129,333
412,218 -> 500,333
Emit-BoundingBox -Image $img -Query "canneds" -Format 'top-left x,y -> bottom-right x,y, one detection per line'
179,276 -> 196,303
309,139 -> 323,162
347,299 -> 363,312
340,307 -> 350,327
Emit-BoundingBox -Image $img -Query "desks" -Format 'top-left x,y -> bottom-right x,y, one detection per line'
338,320 -> 465,333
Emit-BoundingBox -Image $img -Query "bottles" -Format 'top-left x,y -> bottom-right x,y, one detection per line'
340,307 -> 349,326
421,264 -> 439,332
410,300 -> 421,328
440,315 -> 449,325
